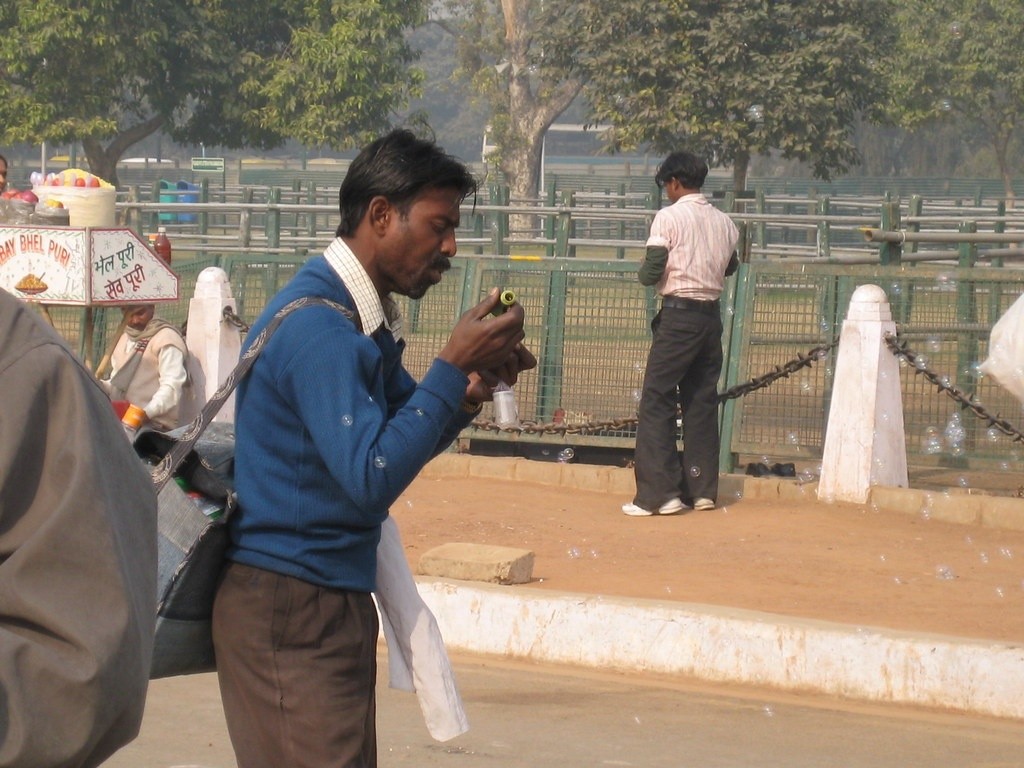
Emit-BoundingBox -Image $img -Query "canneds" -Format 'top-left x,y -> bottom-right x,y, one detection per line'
121,404 -> 146,432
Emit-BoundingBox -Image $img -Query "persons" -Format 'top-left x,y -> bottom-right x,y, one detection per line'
97,304 -> 188,458
211,126 -> 537,768
0,155 -> 7,195
0,287 -> 158,768
622,151 -> 739,517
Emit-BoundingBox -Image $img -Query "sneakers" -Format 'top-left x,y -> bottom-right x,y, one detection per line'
622,497 -> 683,516
680,497 -> 715,511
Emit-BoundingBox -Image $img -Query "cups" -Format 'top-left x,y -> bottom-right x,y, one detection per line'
490,391 -> 519,429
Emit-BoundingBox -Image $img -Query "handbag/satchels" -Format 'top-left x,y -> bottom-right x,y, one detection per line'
132,420 -> 239,680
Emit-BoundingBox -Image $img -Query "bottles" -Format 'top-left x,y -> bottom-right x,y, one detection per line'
120,405 -> 145,444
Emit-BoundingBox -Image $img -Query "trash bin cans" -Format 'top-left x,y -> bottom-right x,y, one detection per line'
175,180 -> 199,224
157,179 -> 176,225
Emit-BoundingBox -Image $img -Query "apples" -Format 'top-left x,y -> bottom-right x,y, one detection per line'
0,187 -> 65,209
53,177 -> 100,188
